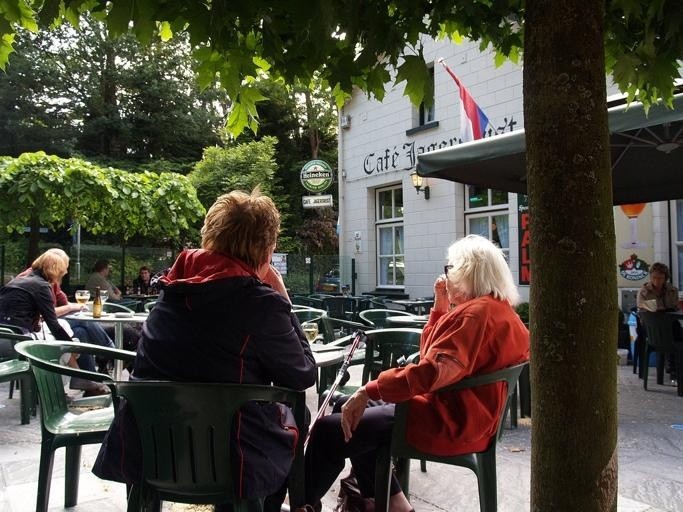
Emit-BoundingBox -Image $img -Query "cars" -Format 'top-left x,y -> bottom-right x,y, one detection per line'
315,263 -> 340,294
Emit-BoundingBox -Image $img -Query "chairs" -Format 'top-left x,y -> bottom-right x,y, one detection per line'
14,338 -> 137,512
102,380 -> 308,512
0,293 -> 159,424
630,307 -> 683,397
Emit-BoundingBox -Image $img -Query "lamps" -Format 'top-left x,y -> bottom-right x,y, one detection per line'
411,165 -> 429,199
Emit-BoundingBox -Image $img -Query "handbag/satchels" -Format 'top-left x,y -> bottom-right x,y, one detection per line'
334,473 -> 377,512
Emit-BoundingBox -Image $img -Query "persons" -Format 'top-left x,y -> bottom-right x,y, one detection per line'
134,266 -> 163,295
0,251 -> 109,391
304,232 -> 530,512
637,263 -> 682,386
14,247 -> 138,397
84,257 -> 122,301
92,184 -> 318,511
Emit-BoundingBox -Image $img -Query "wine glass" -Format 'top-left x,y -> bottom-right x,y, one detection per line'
137,283 -> 141,295
301,322 -> 317,348
126,282 -> 129,295
76,290 -> 90,316
99,291 -> 108,316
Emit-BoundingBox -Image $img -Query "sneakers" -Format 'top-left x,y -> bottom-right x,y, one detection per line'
70,376 -> 99,391
83,385 -> 111,397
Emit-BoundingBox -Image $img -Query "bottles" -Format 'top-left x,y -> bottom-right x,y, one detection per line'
94,287 -> 103,318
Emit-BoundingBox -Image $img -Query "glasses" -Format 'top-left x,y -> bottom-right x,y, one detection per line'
444,265 -> 453,274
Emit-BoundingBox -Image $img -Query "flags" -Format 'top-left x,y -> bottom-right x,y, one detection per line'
448,64 -> 490,143
